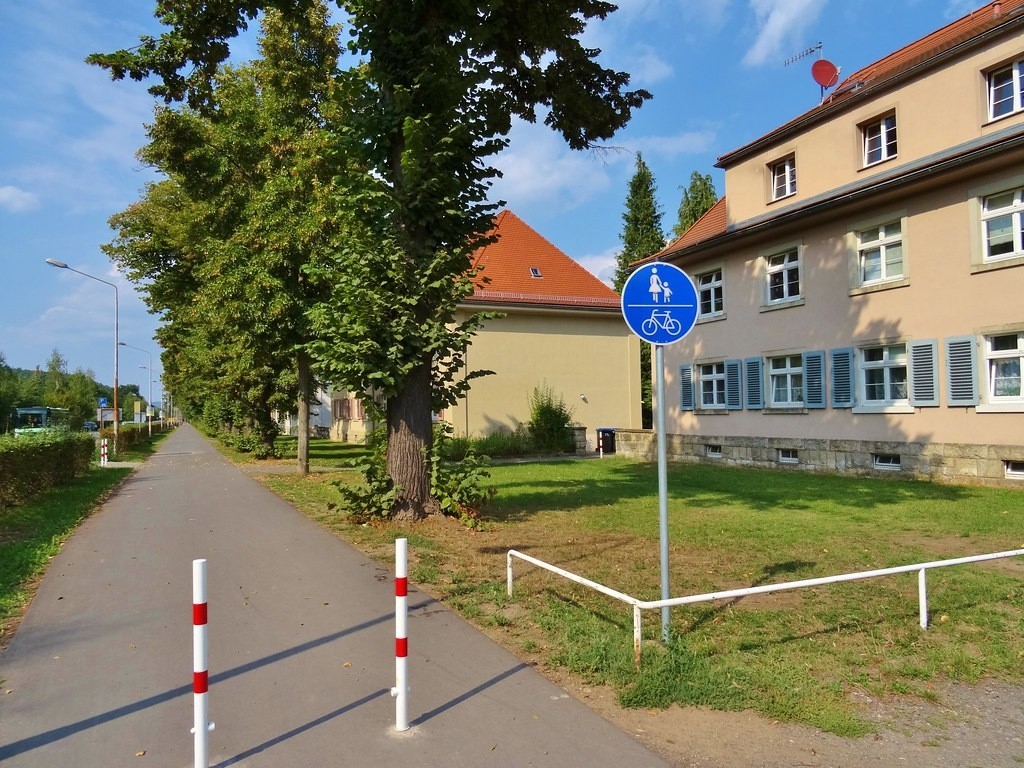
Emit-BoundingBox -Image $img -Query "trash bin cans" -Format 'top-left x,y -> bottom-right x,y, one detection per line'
596,428 -> 616,455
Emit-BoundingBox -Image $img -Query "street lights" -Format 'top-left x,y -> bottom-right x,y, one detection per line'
44,258 -> 121,455
138,365 -> 164,432
154,380 -> 169,430
118,342 -> 152,438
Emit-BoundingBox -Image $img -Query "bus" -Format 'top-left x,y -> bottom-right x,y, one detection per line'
9,406 -> 71,440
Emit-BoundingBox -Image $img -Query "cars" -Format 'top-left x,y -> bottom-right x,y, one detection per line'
81,421 -> 98,432
